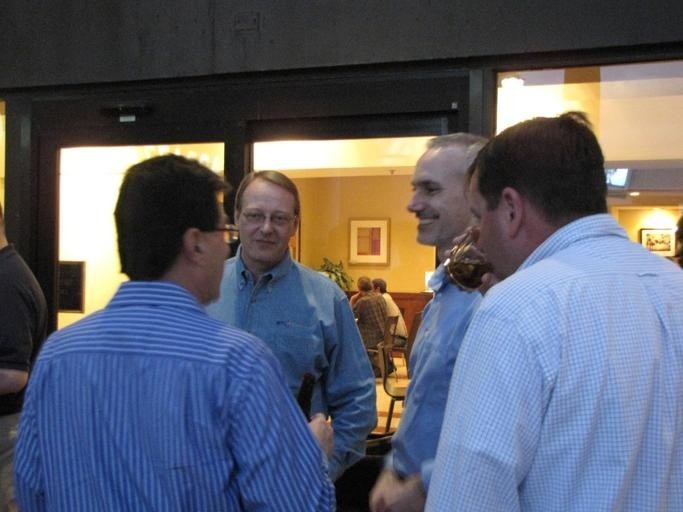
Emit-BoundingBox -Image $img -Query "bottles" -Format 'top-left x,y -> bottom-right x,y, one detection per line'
296,373 -> 315,422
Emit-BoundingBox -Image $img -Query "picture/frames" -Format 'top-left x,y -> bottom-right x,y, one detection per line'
346,218 -> 391,267
639,228 -> 678,258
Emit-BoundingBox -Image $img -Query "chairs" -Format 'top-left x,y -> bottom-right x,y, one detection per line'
377,305 -> 425,432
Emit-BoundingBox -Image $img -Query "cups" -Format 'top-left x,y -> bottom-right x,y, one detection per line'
446,226 -> 498,290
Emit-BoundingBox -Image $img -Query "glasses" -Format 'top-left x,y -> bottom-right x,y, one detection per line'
206,225 -> 239,243
242,211 -> 297,225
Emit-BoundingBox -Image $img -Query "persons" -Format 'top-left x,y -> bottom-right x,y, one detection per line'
0,205 -> 49,512
13,154 -> 337,512
202,170 -> 379,488
666,216 -> 683,269
423,110 -> 683,511
368,133 -> 501,512
350,279 -> 392,376
371,278 -> 405,372
